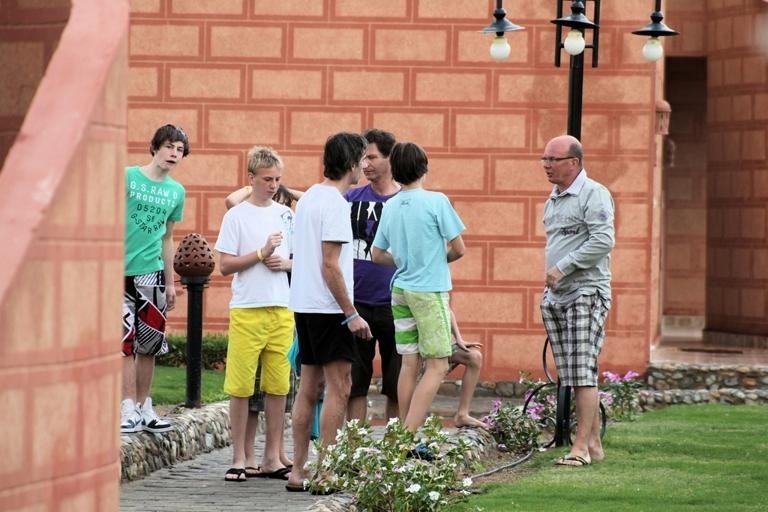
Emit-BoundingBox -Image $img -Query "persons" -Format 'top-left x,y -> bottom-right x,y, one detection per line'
285,127 -> 405,495
449,309 -> 484,427
372,142 -> 467,461
120,124 -> 191,432
216,147 -> 306,481
541,134 -> 617,468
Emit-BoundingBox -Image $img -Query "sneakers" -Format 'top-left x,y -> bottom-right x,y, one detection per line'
119,398 -> 148,434
136,396 -> 174,434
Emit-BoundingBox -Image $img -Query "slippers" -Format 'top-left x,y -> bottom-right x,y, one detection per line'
285,484 -> 311,492
406,441 -> 435,462
312,488 -> 341,496
244,466 -> 266,478
554,455 -> 588,467
224,468 -> 246,482
258,466 -> 291,480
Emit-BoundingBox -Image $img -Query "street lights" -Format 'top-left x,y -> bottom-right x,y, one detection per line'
480,0 -> 679,448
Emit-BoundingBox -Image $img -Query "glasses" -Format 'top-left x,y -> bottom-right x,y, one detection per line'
540,156 -> 575,162
